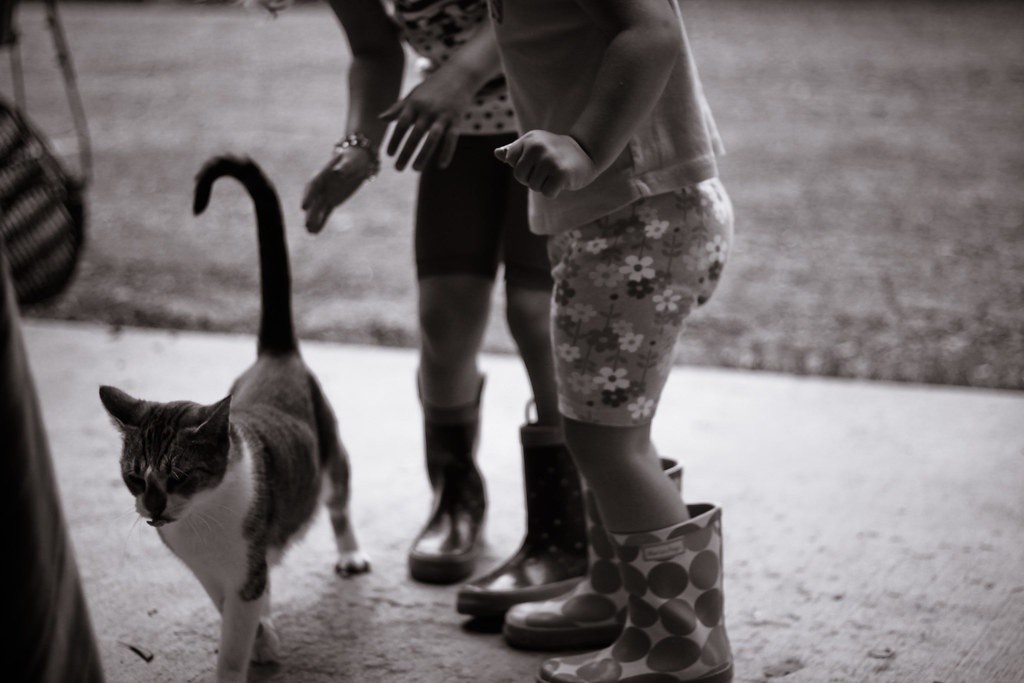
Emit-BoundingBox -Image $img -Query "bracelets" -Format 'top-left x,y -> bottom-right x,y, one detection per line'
334,131 -> 381,183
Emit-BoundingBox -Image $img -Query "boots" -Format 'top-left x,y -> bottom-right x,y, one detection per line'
456,422 -> 588,614
535,504 -> 733,683
410,367 -> 485,582
503,456 -> 684,650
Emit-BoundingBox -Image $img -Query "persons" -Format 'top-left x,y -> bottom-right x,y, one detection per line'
300,0 -> 595,615
489,0 -> 736,683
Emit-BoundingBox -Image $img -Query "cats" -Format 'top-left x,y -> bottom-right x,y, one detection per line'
99,154 -> 372,682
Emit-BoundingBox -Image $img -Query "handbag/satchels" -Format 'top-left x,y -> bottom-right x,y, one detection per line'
0,0 -> 93,308
